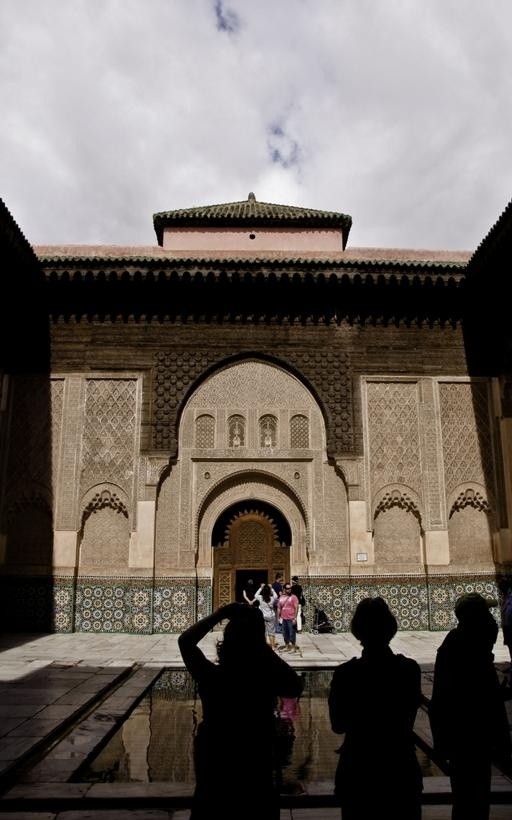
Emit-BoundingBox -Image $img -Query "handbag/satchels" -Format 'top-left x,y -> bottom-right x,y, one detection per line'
264,609 -> 277,621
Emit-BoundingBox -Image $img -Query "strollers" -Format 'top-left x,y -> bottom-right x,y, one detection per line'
309,604 -> 337,635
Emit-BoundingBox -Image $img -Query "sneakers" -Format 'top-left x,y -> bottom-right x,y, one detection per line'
272,644 -> 297,654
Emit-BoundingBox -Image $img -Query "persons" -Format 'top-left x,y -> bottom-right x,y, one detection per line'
174,602 -> 307,820
240,571 -> 304,655
426,592 -> 512,819
277,695 -> 299,730
325,594 -> 427,820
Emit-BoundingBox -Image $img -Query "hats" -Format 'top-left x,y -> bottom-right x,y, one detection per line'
455,593 -> 498,616
